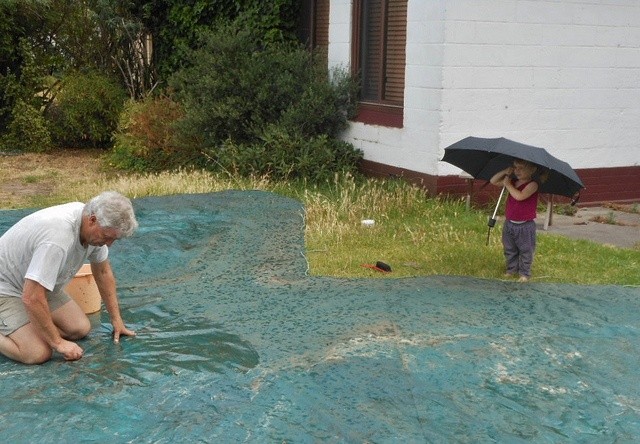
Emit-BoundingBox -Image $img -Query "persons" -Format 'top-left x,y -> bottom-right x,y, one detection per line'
490,158 -> 538,282
0,191 -> 139,364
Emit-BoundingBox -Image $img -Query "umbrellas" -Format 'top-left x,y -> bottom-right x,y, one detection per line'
438,135 -> 585,246
